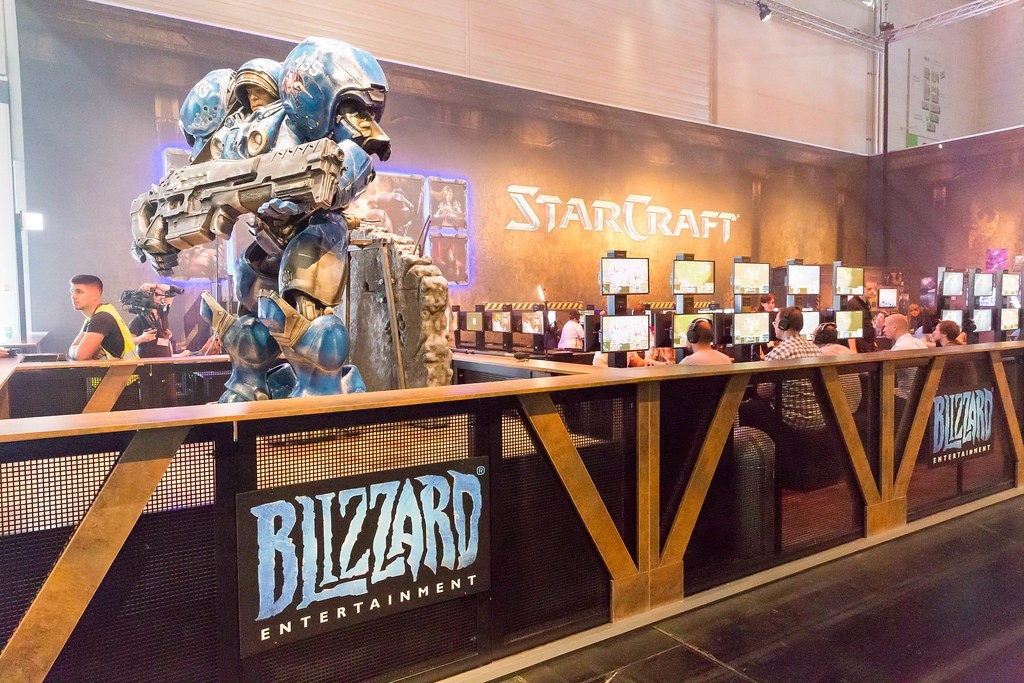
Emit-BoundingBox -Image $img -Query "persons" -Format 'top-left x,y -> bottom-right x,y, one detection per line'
129,284 -> 184,408
935,320 -> 976,403
758,308 -> 862,446
130,37 -> 391,405
884,315 -> 930,414
758,286 -> 926,336
68,274 -> 140,408
680,318 -> 732,364
558,311 -> 584,350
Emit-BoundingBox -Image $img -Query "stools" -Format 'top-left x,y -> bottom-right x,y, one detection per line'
783,422 -> 847,493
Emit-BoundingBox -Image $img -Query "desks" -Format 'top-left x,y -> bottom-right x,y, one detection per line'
0,413 -> 613,683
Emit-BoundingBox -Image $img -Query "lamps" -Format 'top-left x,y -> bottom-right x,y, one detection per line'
13,210 -> 44,230
757,1 -> 773,21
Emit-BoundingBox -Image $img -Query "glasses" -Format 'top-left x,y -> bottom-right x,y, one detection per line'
773,321 -> 778,325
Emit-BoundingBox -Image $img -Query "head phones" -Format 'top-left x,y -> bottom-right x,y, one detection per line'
815,323 -> 837,344
778,306 -> 801,331
687,318 -> 712,343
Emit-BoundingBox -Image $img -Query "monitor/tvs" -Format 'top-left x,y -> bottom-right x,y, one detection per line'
672,259 -> 865,349
600,257 -> 649,295
601,314 -> 650,353
942,271 -> 1021,333
878,288 -> 897,308
450,310 -> 594,335
